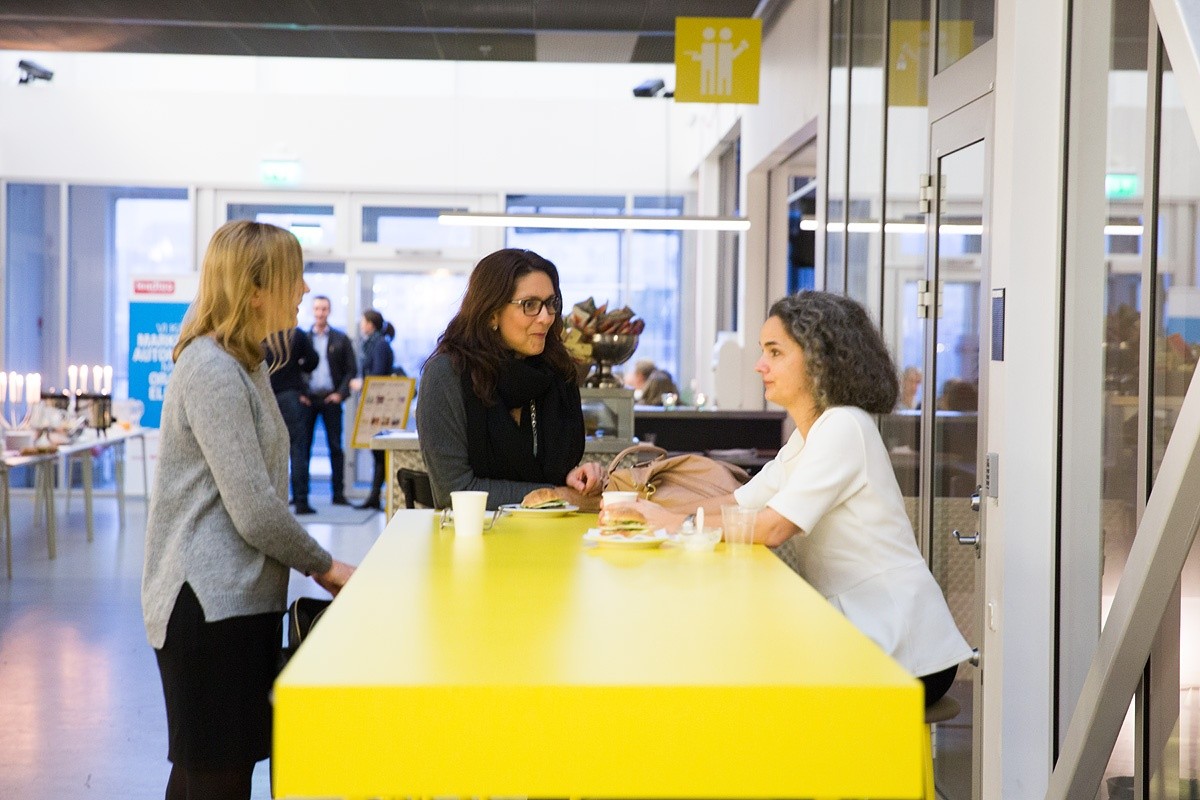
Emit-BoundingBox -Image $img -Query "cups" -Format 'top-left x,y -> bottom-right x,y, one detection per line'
720,504 -> 760,553
662,393 -> 677,410
450,491 -> 489,537
602,491 -> 638,517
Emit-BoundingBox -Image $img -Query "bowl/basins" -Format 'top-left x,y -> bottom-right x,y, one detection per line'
6,435 -> 32,450
677,526 -> 722,549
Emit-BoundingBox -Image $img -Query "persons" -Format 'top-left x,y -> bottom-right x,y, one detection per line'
901,365 -> 976,411
415,249 -> 606,514
625,360 -> 688,406
598,290 -> 974,708
261,326 -> 318,514
290,295 -> 357,505
349,310 -> 395,508
139,220 -> 357,800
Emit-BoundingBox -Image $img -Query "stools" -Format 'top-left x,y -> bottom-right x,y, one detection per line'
925,695 -> 960,723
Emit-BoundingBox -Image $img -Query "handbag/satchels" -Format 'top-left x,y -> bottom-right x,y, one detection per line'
602,444 -> 749,508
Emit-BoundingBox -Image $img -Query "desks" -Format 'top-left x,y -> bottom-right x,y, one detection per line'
272,510 -> 939,799
0,428 -> 150,582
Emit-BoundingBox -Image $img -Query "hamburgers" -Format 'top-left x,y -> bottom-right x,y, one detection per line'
599,507 -> 647,538
521,488 -> 566,509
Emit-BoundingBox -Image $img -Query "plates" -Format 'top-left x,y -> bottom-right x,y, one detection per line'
584,533 -> 669,549
498,504 -> 579,517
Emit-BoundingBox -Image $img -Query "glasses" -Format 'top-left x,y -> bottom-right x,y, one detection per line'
508,296 -> 561,316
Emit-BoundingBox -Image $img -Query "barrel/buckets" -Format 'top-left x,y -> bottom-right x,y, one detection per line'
77,394 -> 111,429
42,393 -> 68,412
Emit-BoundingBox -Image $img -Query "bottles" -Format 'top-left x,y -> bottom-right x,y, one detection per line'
708,331 -> 743,411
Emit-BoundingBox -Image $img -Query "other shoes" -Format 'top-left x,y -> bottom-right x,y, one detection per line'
296,501 -> 316,514
332,496 -> 350,505
356,499 -> 380,509
